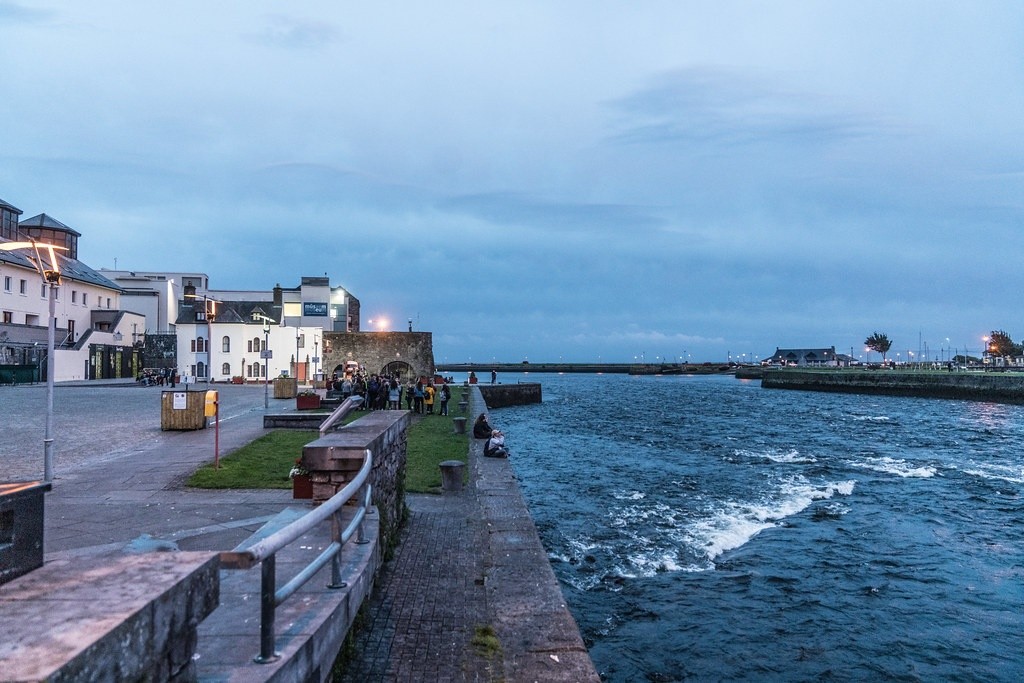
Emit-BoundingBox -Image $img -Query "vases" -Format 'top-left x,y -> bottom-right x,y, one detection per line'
293,475 -> 313,499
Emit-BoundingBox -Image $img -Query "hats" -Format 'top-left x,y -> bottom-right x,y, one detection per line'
492,430 -> 500,438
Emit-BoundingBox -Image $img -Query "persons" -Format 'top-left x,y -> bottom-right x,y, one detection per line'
150,366 -> 176,388
325,370 -> 451,416
473,412 -> 493,439
488,430 -> 509,458
491,369 -> 496,384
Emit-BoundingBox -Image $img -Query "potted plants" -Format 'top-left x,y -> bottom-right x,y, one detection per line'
273,374 -> 297,398
292,389 -> 320,410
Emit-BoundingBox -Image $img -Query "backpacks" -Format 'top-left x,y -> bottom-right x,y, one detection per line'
484,438 -> 492,456
424,387 -> 432,400
440,391 -> 446,401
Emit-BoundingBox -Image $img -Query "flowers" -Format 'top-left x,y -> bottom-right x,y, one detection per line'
289,458 -> 310,480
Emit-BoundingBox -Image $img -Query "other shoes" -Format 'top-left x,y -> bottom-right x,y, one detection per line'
438,413 -> 442,415
445,414 -> 447,416
363,407 -> 365,411
358,407 -> 361,410
